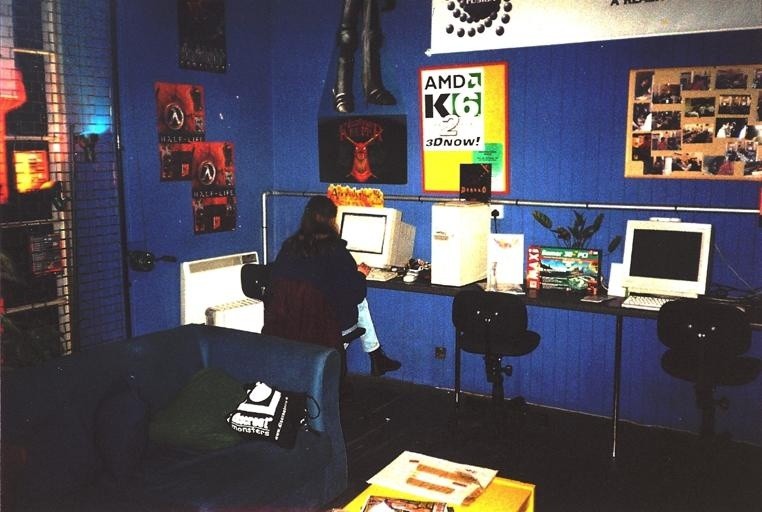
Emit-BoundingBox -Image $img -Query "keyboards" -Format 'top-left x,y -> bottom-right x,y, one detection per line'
365,270 -> 398,282
621,296 -> 675,312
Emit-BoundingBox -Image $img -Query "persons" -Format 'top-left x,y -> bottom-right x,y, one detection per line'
631,70 -> 762,177
273,194 -> 401,375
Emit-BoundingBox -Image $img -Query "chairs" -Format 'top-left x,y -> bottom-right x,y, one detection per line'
451,288 -> 541,407
653,295 -> 762,458
240,261 -> 370,404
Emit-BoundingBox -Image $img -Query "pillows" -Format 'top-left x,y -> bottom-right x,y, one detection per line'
147,366 -> 252,451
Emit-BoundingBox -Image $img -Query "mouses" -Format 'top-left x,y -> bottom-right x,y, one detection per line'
403,275 -> 416,282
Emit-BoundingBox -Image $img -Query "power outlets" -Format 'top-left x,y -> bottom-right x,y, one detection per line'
489,204 -> 506,221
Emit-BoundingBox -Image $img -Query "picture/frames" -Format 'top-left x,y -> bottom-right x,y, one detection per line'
418,60 -> 509,195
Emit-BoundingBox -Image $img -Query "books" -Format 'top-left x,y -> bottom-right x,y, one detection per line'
359,495 -> 453,512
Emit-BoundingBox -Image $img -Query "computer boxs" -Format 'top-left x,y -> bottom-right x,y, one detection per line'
430,203 -> 490,287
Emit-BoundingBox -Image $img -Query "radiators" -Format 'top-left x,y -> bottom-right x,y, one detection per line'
180,250 -> 261,327
206,296 -> 265,333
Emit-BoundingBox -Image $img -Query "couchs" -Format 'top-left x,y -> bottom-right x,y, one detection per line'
1,321 -> 351,511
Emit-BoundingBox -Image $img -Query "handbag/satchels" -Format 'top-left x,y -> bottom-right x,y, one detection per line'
225,380 -> 322,450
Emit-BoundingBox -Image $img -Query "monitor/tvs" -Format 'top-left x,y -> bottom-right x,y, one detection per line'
335,206 -> 416,270
622,220 -> 712,299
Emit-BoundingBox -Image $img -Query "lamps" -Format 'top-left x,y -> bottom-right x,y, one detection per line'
40,123 -> 101,355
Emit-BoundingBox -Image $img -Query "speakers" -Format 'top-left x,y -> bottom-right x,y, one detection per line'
607,262 -> 630,297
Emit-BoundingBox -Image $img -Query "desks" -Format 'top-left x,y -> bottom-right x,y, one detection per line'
339,467 -> 542,511
360,263 -> 761,460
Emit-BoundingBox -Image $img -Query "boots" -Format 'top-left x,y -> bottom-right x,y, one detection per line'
369,345 -> 401,377
